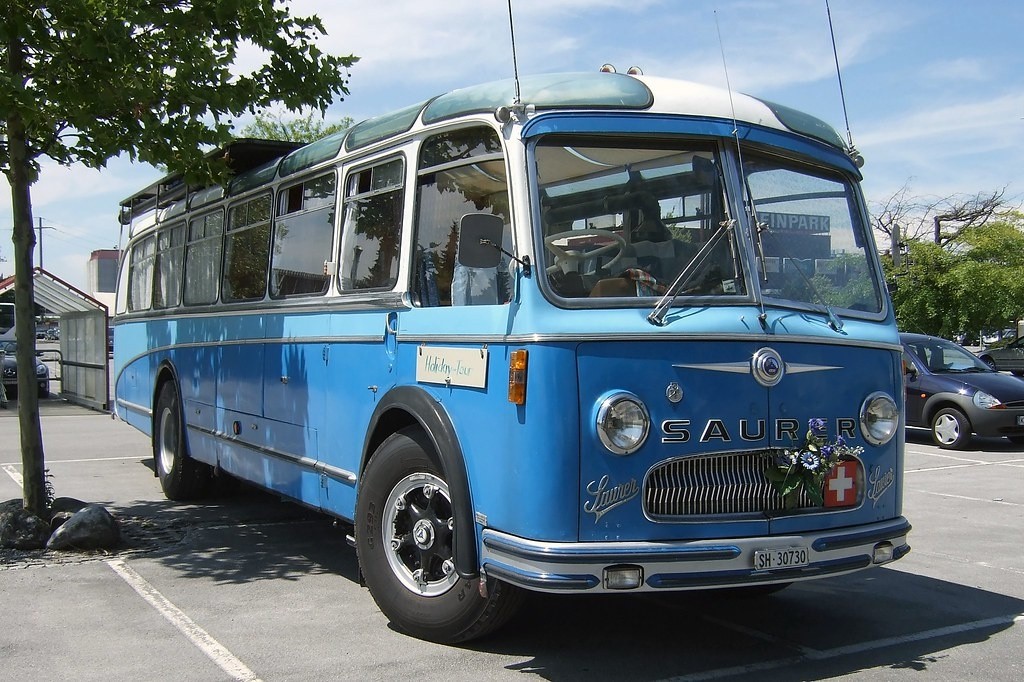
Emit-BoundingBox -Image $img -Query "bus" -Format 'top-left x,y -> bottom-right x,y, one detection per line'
107,62 -> 914,647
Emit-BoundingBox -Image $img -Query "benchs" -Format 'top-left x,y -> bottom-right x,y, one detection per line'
223,226 -> 734,307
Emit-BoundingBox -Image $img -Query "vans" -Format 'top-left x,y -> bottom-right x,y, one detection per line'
0,303 -> 17,343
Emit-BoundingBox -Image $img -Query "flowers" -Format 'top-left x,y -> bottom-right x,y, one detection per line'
762,418 -> 865,520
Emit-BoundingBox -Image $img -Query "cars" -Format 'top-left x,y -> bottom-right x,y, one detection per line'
983,329 -> 1017,350
36,328 -> 59,341
977,336 -> 1024,376
108,326 -> 115,348
899,332 -> 1024,452
1,340 -> 50,401
